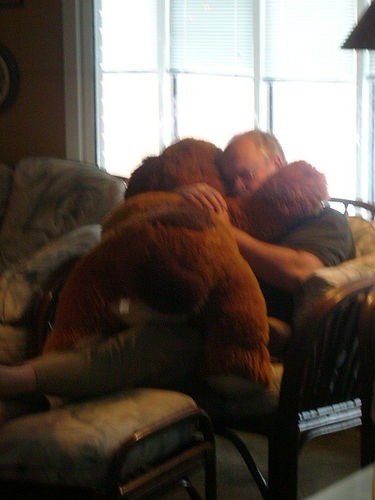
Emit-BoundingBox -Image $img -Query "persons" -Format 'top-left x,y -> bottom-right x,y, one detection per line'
1,131 -> 355,423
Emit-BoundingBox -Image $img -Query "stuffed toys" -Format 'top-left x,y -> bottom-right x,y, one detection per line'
43,138 -> 329,410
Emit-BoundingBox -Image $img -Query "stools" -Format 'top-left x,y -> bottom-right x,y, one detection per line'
0,388 -> 217,500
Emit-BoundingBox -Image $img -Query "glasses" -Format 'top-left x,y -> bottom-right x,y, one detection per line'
222,161 -> 259,188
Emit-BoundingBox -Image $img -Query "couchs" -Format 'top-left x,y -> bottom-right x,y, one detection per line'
42,160 -> 375,500
0,157 -> 129,362
